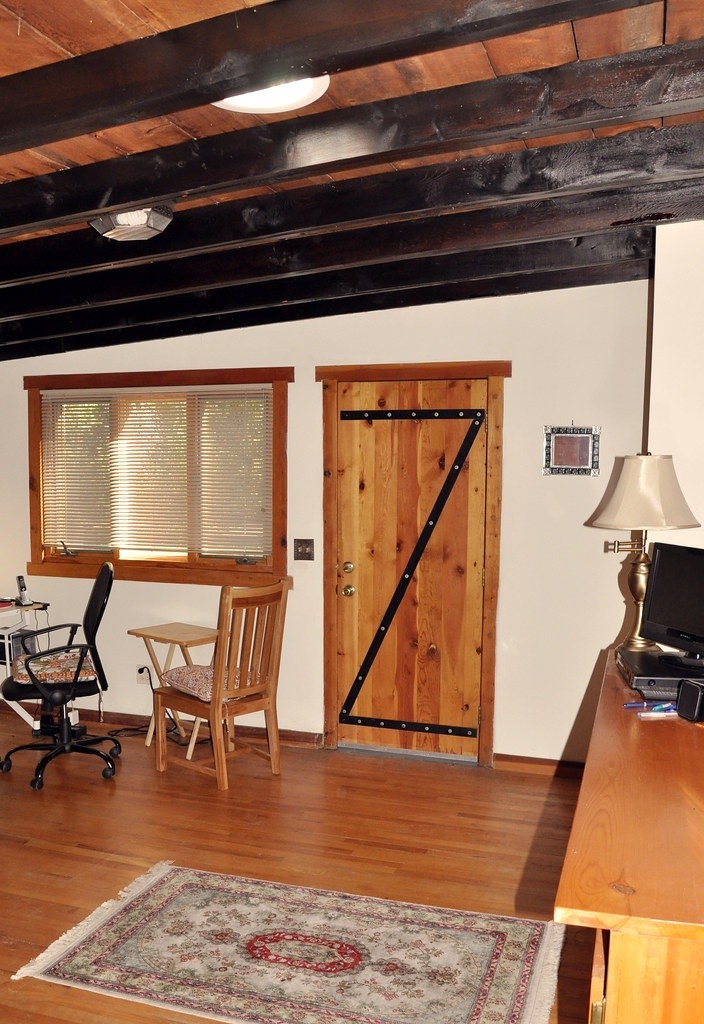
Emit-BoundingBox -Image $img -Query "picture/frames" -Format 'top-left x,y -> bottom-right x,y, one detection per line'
544,425 -> 600,476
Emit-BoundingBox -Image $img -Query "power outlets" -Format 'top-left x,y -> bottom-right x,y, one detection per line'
135,664 -> 154,684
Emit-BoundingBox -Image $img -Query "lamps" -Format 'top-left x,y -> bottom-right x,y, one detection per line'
90,206 -> 174,242
585,453 -> 702,653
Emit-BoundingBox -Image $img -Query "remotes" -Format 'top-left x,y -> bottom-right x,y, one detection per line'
636,685 -> 679,701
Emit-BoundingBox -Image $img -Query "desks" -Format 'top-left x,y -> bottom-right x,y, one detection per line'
554,645 -> 704,1021
0,598 -> 80,732
127,622 -> 232,760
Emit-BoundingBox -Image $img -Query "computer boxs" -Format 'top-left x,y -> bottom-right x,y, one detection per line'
614,650 -> 704,690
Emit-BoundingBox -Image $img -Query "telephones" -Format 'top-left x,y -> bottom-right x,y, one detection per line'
15,576 -> 34,606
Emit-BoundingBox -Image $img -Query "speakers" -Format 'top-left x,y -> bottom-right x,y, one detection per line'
677,680 -> 704,722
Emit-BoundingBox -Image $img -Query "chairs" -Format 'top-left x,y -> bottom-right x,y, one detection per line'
0,563 -> 122,791
154,579 -> 292,789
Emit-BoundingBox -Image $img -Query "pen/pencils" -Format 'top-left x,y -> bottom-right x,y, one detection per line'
622,702 -> 678,719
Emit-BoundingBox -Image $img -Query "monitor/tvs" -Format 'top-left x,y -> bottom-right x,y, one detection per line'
638,542 -> 704,671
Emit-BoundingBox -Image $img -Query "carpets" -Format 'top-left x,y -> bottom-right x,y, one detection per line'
11,855 -> 565,1023
